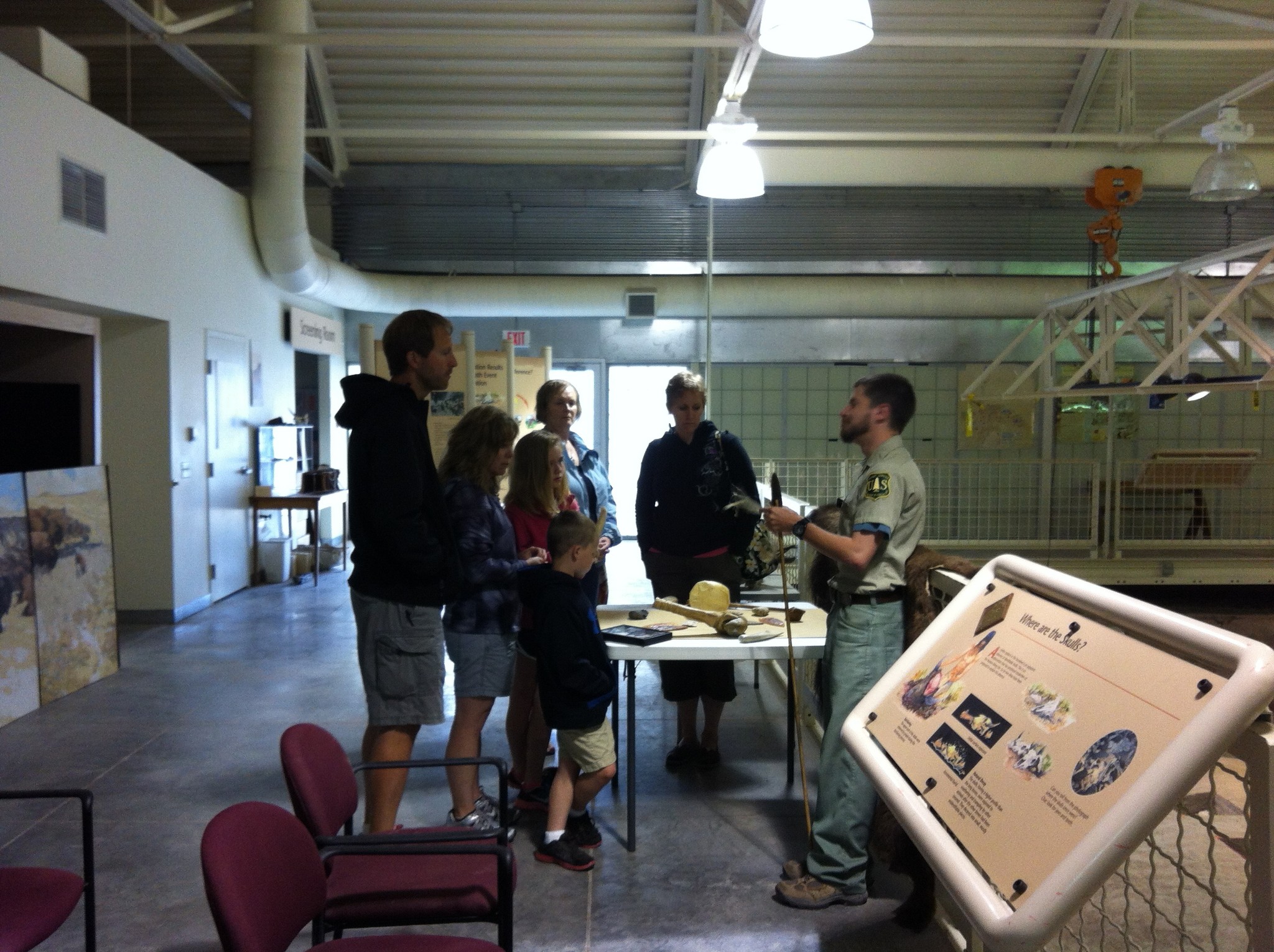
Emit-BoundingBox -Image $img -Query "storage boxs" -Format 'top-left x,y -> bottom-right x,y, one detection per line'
0,26 -> 92,104
291,545 -> 338,577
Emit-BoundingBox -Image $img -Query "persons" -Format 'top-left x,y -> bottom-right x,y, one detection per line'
441,379 -> 618,870
635,373 -> 760,768
761,373 -> 928,907
335,309 -> 457,834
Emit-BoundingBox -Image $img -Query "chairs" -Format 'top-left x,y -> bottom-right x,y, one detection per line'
0,789 -> 96,952
199,721 -> 517,952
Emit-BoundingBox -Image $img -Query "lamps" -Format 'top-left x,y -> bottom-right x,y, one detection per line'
1147,372 -> 1212,410
1188,101 -> 1261,204
696,96 -> 767,200
757,0 -> 874,58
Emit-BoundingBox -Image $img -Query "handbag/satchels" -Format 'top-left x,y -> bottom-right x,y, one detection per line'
735,519 -> 781,581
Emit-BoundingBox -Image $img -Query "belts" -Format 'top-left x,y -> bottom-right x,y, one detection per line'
850,591 -> 905,606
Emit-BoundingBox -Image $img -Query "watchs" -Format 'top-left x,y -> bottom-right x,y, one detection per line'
792,517 -> 812,540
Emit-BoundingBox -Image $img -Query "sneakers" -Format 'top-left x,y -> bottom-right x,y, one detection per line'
567,812 -> 602,848
473,785 -> 502,823
783,860 -> 809,879
445,805 -> 516,841
776,875 -> 868,909
535,834 -> 595,870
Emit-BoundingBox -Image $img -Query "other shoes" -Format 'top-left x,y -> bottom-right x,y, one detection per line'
507,766 -> 557,789
696,746 -> 721,769
515,786 -> 549,808
664,745 -> 696,770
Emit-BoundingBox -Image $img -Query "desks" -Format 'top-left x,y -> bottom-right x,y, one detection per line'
248,490 -> 350,589
604,599 -> 831,853
739,573 -> 803,688
